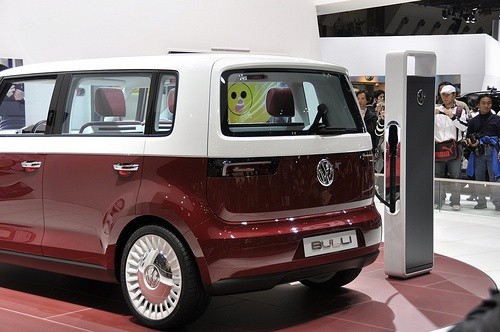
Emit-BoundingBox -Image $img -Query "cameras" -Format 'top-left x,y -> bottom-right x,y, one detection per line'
468,132 -> 481,142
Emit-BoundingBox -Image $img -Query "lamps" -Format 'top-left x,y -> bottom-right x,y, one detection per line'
471,17 -> 477,24
465,16 -> 471,24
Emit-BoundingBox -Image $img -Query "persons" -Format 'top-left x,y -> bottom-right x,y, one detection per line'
3,83 -> 25,103
437,82 -> 500,211
354,88 -> 385,174
434,85 -> 468,210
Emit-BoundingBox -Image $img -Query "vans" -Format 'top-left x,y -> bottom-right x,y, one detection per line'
0,51 -> 383,332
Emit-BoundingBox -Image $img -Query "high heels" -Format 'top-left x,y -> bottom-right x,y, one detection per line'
467,195 -> 477,201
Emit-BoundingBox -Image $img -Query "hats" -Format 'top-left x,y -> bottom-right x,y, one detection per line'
440,85 -> 456,94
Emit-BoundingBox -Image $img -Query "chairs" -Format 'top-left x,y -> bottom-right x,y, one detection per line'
168,88 -> 176,115
266,87 -> 295,123
94,87 -> 126,122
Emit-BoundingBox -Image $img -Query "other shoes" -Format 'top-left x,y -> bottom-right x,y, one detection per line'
437,200 -> 444,209
452,205 -> 460,211
495,206 -> 500,211
474,202 -> 487,209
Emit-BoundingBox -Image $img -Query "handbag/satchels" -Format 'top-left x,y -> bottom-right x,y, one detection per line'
435,139 -> 457,163
